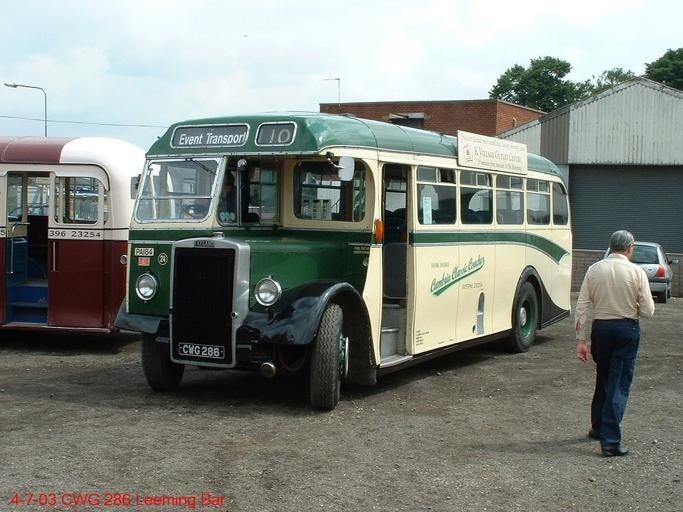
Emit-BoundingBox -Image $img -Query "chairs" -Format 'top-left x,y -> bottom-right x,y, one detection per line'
321,206 -> 572,227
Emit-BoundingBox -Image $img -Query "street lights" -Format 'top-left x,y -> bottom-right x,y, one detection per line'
3,81 -> 47,137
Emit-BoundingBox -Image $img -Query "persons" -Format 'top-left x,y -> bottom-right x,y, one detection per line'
212,170 -> 249,213
571,227 -> 657,459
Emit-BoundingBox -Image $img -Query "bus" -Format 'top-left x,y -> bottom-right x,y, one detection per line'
110,111 -> 577,413
0,135 -> 178,346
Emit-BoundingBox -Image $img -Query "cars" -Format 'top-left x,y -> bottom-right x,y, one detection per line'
601,240 -> 680,305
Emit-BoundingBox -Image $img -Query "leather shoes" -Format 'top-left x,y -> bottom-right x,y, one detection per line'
589,428 -> 628,456
